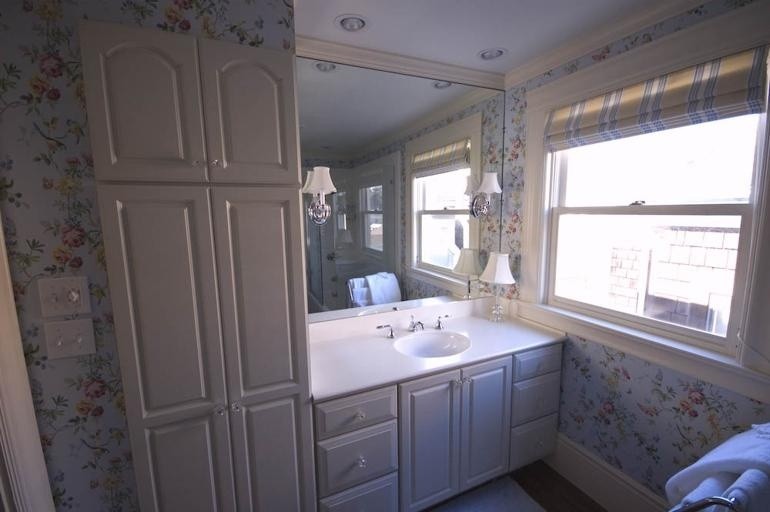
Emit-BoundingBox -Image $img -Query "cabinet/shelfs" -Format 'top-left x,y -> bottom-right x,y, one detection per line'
126,384 -> 314,510
314,344 -> 563,512
82,26 -> 303,189
92,182 -> 312,420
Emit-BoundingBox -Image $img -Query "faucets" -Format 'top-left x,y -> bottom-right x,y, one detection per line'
393,305 -> 398,310
408,315 -> 425,332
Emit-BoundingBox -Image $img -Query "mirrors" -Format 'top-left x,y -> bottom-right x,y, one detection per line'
294,39 -> 504,325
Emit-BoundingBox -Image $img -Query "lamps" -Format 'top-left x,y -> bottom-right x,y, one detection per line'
300,165 -> 337,225
453,249 -> 483,300
478,252 -> 517,324
472,173 -> 502,217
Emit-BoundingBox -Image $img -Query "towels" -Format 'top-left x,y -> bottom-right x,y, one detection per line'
346,271 -> 402,308
664,418 -> 768,512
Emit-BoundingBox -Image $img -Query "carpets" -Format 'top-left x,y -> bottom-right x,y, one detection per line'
429,474 -> 546,512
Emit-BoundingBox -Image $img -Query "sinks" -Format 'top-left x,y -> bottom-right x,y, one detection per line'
395,330 -> 472,359
360,305 -> 408,314
